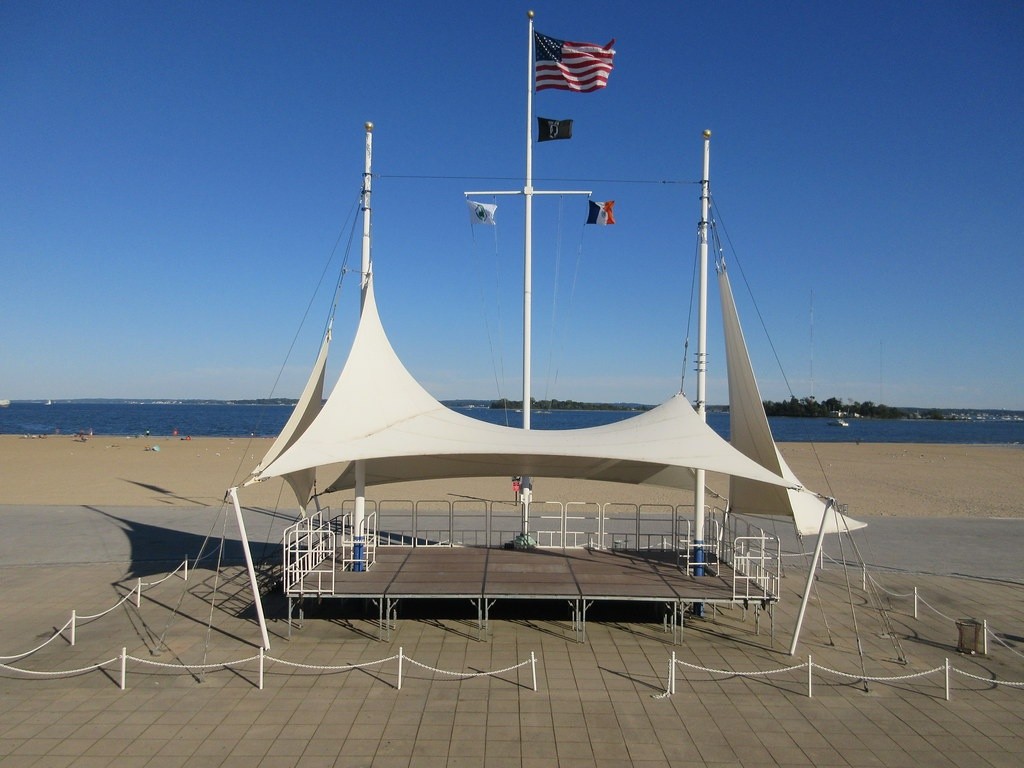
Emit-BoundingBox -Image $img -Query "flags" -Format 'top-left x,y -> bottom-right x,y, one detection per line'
468,200 -> 497,224
535,31 -> 616,92
586,200 -> 615,225
537,117 -> 572,143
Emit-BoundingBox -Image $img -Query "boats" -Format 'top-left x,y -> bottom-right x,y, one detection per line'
827,419 -> 848,427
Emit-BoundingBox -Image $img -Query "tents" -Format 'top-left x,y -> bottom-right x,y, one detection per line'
229,263 -> 868,655
